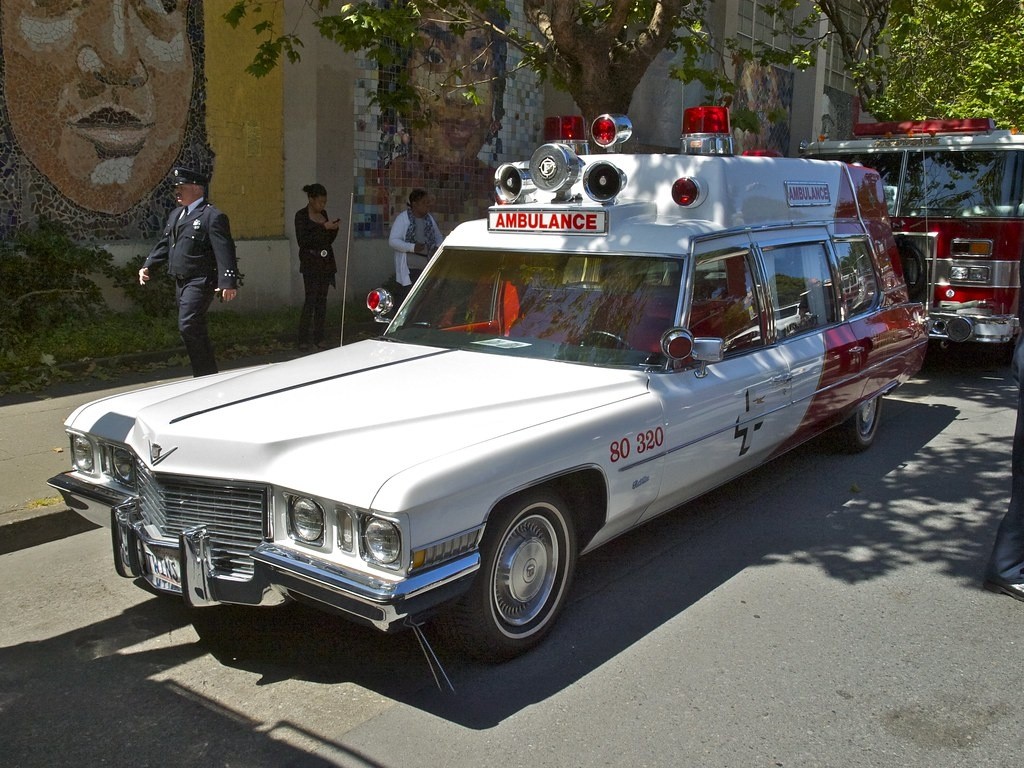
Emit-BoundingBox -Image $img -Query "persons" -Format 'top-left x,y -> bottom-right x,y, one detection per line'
389,189 -> 443,299
983,331 -> 1024,603
139,167 -> 239,377
294,183 -> 341,352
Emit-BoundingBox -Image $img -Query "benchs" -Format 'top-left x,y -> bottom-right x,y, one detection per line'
507,278 -> 753,364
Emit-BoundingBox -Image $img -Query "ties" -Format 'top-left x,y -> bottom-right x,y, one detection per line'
178,206 -> 188,223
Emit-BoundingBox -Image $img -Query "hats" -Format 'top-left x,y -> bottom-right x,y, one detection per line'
170,167 -> 208,186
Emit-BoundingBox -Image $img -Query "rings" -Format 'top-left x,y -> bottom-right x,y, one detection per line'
231,294 -> 233,295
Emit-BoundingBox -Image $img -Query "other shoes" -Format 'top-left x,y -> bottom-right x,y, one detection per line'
298,341 -> 308,351
984,576 -> 1024,603
315,339 -> 331,350
